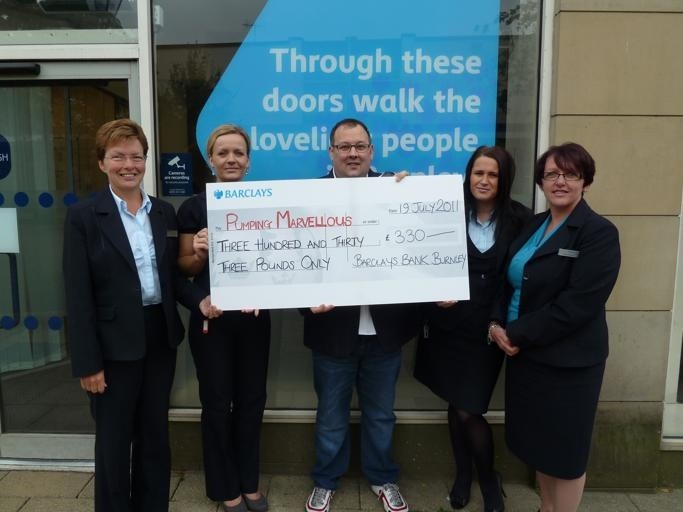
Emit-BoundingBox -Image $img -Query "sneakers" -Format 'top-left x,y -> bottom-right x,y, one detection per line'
370,483 -> 408,512
305,487 -> 336,512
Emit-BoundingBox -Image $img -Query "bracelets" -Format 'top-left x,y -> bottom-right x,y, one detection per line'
487,324 -> 499,345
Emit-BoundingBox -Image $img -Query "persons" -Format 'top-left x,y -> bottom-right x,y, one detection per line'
298,118 -> 422,512
62,120 -> 226,512
412,144 -> 534,512
486,142 -> 621,512
177,123 -> 276,512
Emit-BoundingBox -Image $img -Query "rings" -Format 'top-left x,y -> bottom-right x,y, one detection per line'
197,233 -> 200,237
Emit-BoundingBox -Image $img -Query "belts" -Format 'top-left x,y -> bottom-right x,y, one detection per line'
143,304 -> 161,312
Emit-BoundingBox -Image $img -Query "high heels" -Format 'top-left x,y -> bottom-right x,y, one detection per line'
450,473 -> 473,510
478,472 -> 507,512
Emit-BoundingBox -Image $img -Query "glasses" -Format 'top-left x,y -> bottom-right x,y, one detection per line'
541,171 -> 583,181
330,143 -> 370,151
101,154 -> 144,161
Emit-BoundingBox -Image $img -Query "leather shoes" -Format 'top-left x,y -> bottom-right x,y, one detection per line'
242,492 -> 268,512
222,495 -> 248,512
